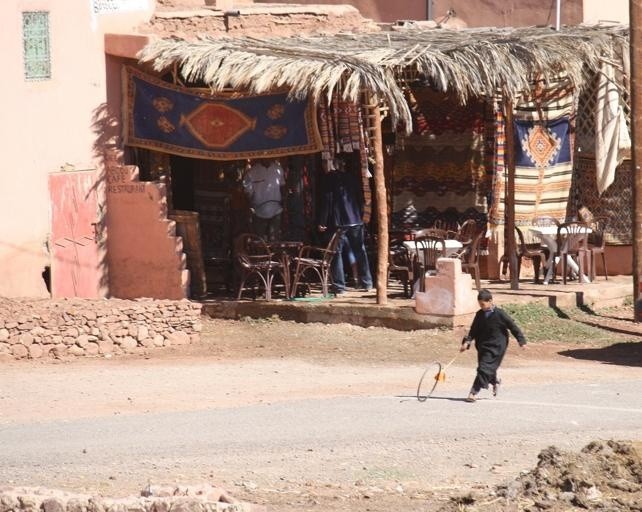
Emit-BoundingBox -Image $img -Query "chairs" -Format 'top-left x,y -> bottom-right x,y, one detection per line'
494,225 -> 547,281
532,218 -> 561,275
586,216 -> 609,283
205,216 -> 341,303
554,220 -> 587,286
386,218 -> 487,297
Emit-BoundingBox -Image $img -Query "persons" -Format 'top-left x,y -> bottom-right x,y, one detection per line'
460,290 -> 528,402
317,157 -> 375,293
242,157 -> 287,241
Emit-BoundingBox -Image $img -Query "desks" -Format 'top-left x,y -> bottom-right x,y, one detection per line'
528,225 -> 594,285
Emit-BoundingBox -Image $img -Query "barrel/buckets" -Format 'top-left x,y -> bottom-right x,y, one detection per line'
167,209 -> 207,300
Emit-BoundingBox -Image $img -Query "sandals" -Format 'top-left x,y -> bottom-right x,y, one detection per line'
493,378 -> 501,398
466,394 -> 475,402
